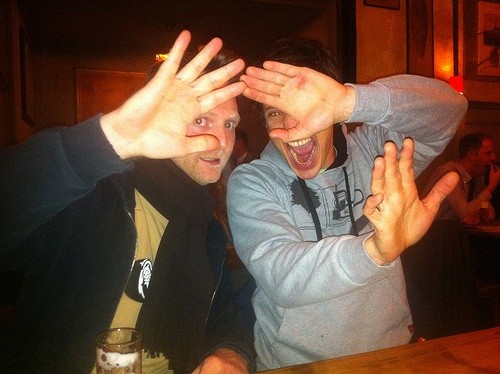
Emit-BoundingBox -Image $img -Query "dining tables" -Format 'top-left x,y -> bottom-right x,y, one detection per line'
460,221 -> 500,309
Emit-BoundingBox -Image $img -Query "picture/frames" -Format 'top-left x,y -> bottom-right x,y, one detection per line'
364,0 -> 401,10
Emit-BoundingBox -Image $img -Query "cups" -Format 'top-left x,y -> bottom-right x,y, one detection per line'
95,327 -> 142,374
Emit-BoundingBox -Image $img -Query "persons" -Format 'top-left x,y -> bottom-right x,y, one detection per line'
421,134 -> 500,225
225,38 -> 468,374
0,30 -> 257,374
218,125 -> 261,182
